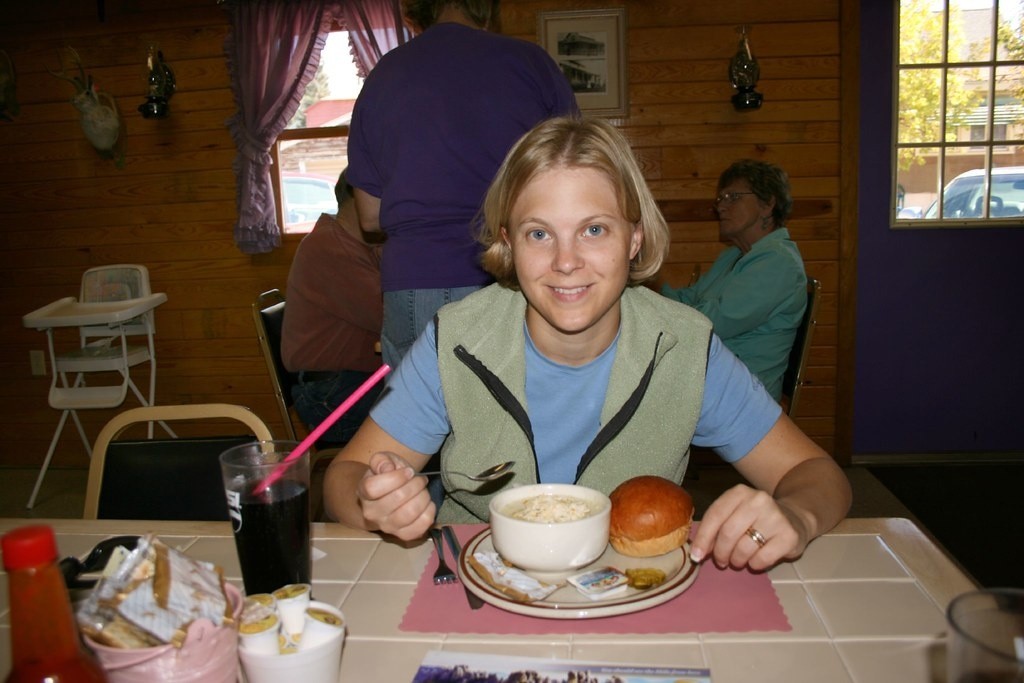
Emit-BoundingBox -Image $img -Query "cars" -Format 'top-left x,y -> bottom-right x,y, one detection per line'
282,170 -> 339,234
898,165 -> 1024,220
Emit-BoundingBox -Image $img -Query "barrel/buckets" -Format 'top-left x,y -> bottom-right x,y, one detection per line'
238,601 -> 346,683
81,582 -> 243,683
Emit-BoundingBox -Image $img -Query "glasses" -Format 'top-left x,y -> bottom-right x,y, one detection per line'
714,192 -> 756,208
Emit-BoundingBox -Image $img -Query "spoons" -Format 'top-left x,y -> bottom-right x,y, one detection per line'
415,461 -> 516,480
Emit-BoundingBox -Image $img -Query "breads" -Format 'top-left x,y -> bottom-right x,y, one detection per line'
607,475 -> 695,558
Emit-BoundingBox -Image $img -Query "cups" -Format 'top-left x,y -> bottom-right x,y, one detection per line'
237,584 -> 345,657
220,439 -> 312,596
946,588 -> 1024,683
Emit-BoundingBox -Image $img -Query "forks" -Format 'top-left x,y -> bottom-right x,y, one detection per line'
428,528 -> 457,585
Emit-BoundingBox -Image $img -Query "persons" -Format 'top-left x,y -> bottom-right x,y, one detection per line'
346,0 -> 587,516
659,159 -> 809,523
323,118 -> 852,573
281,167 -> 385,454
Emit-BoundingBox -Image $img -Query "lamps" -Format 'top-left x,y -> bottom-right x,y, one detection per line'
727,24 -> 767,113
135,42 -> 179,121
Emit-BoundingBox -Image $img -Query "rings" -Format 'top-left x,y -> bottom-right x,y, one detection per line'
746,527 -> 765,548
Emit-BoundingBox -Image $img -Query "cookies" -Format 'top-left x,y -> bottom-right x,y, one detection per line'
468,553 -> 558,602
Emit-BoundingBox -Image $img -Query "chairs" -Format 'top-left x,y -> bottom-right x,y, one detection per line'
82,404 -> 280,522
251,287 -> 347,524
686,276 -> 825,522
55,262 -> 156,373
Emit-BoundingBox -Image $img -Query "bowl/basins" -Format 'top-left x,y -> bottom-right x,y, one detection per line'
489,483 -> 612,578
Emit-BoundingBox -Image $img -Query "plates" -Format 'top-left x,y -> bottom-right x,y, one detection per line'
457,527 -> 701,618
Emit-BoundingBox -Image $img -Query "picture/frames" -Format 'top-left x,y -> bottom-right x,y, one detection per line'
534,9 -> 631,121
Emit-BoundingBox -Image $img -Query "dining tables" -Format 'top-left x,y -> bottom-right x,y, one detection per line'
0,515 -> 995,683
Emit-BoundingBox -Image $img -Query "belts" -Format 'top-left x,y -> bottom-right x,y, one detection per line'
291,370 -> 332,385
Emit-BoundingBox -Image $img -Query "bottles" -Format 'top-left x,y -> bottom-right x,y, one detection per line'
0,524 -> 107,683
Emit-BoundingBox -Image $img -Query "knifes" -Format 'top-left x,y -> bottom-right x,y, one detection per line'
441,525 -> 485,609
